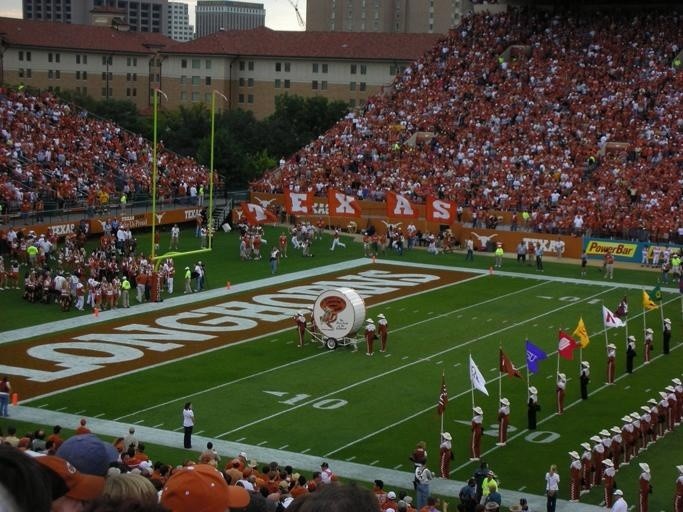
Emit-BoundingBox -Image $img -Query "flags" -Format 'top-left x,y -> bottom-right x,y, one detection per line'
612,296 -> 631,317
499,346 -> 525,380
526,342 -> 548,374
600,303 -> 627,328
572,317 -> 591,348
556,330 -> 577,361
469,353 -> 489,397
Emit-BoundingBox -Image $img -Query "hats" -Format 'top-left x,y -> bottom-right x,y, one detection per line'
384,491 -> 413,512
7,419 -> 145,456
55,435 -> 121,476
32,456 -> 107,502
239,452 -> 329,490
298,305 -> 386,322
161,465 -> 250,512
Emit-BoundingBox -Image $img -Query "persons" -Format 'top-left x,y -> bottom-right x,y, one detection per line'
293,313 -> 306,348
363,318 -> 375,356
605,343 -> 616,384
1,375 -> 683,512
626,334 -> 637,375
306,313 -> 318,343
377,313 -> 388,352
642,327 -> 654,367
664,317 -> 672,354
228,0 -> 683,282
580,359 -> 592,399
1,89 -> 225,311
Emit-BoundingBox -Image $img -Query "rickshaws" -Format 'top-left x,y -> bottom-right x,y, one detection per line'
304,327 -> 368,349
